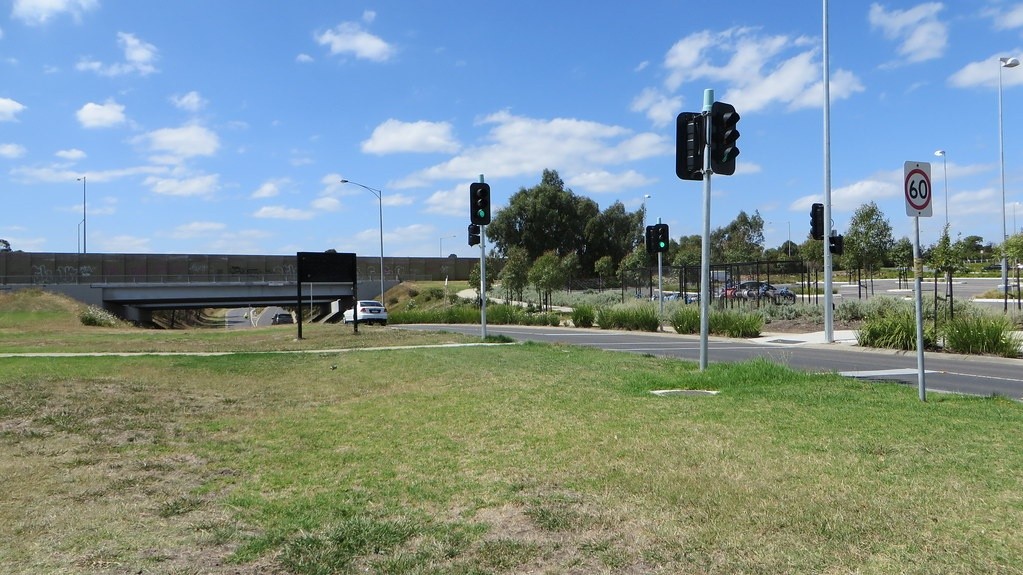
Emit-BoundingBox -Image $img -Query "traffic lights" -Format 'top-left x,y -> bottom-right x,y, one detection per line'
654,223 -> 670,253
470,183 -> 492,224
707,101 -> 740,175
830,235 -> 844,255
808,203 -> 825,240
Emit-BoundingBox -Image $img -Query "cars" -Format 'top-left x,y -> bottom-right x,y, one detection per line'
982,264 -> 1010,270
271,313 -> 294,325
342,299 -> 387,327
729,282 -> 797,306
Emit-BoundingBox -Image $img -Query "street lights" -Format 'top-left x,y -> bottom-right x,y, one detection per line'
642,194 -> 651,252
77,177 -> 88,254
339,179 -> 385,306
77,218 -> 85,254
997,55 -> 1021,314
936,149 -> 948,231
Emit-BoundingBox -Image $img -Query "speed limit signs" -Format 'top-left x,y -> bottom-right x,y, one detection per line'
903,160 -> 935,217
